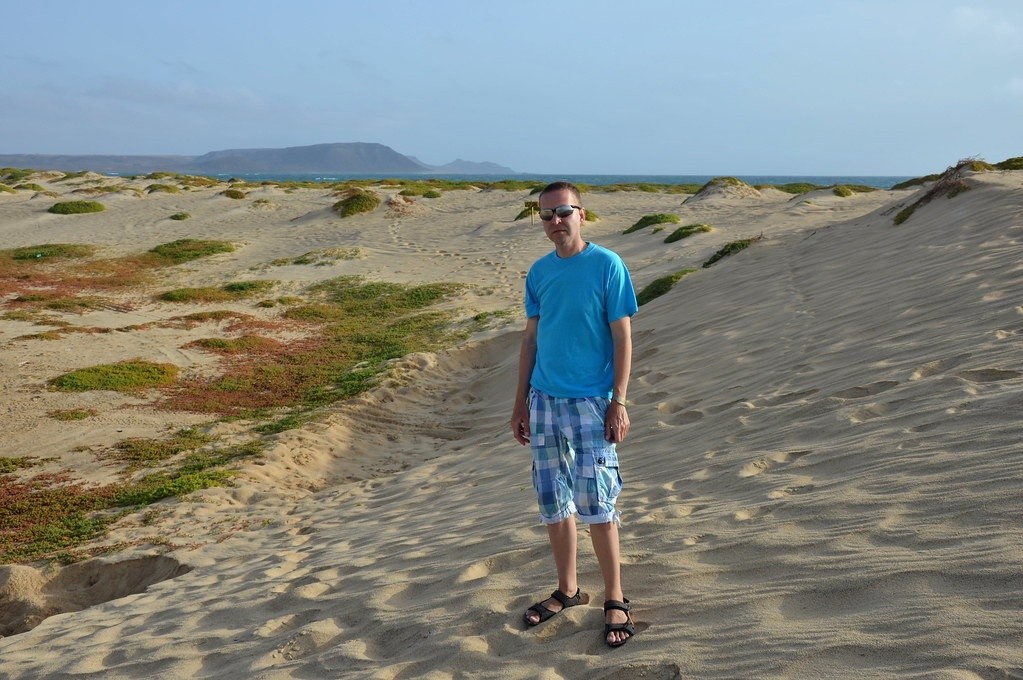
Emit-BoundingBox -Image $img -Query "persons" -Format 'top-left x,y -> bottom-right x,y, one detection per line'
510,181 -> 638,647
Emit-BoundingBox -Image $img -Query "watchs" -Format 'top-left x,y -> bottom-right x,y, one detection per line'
611,395 -> 626,407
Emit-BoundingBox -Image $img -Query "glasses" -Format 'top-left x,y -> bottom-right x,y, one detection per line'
538,205 -> 581,221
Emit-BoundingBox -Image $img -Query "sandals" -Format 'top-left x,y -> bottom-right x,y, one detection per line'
604,597 -> 635,648
522,587 -> 581,625
